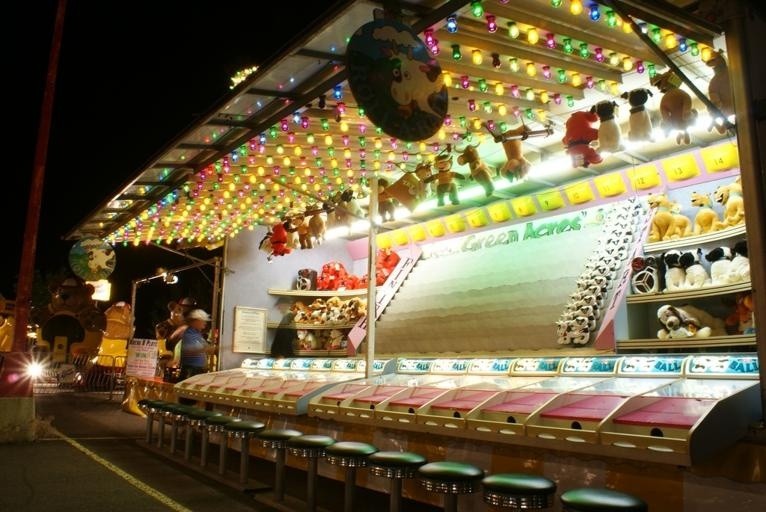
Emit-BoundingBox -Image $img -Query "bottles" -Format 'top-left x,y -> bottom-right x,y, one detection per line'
187,308 -> 212,322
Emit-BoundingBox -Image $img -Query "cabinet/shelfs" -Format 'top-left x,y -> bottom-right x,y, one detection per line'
616,222 -> 758,351
265,288 -> 367,357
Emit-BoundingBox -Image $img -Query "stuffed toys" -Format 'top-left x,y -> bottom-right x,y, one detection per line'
456,144 -> 496,198
690,192 -> 720,235
562,111 -> 602,168
316,261 -> 369,291
500,126 -> 531,183
423,155 -> 466,206
646,194 -> 693,242
661,248 -> 713,292
290,204 -> 326,249
288,296 -> 367,351
708,54 -> 736,134
324,189 -> 363,228
653,76 -> 698,147
590,100 -> 625,153
620,88 -> 656,143
408,163 -> 439,200
372,177 -> 396,223
707,239 -> 751,285
376,248 -> 400,285
267,221 -> 293,262
725,291 -> 755,335
657,304 -> 728,339
714,176 -> 745,230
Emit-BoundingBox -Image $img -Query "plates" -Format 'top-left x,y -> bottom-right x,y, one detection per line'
616,222 -> 758,351
265,288 -> 367,357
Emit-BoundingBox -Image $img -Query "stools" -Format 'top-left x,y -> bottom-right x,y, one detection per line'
266,428 -> 428,511
136,400 -> 266,500
415,459 -> 650,511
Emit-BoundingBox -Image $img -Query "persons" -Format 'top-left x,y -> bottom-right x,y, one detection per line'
176,311 -> 216,408
